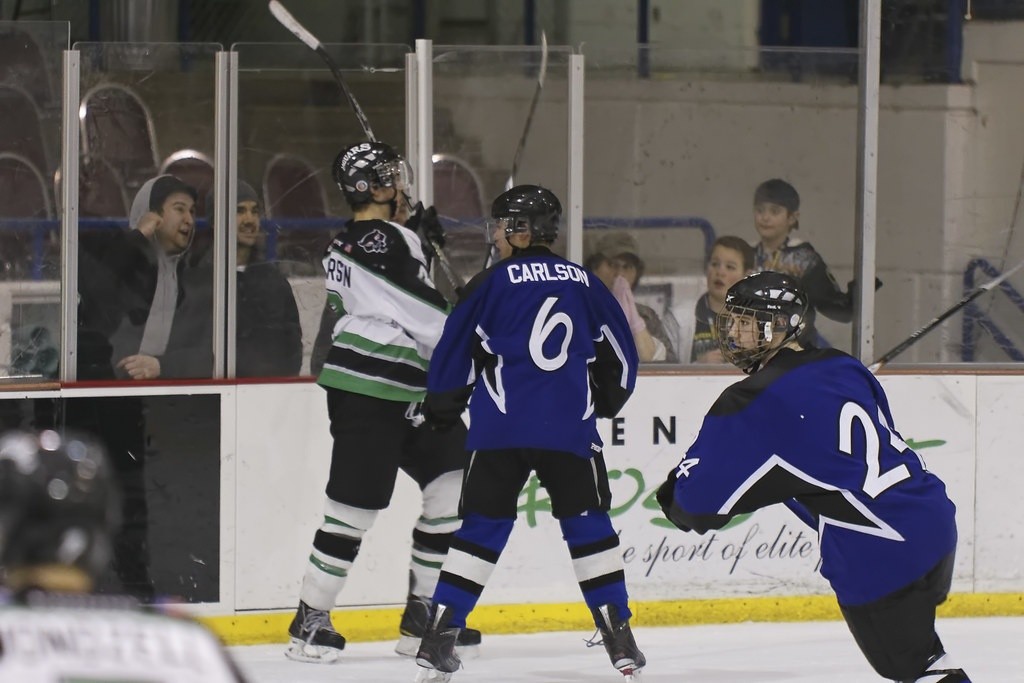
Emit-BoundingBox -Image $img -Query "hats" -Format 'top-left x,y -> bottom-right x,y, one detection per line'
203,176 -> 260,221
595,232 -> 641,261
757,179 -> 800,230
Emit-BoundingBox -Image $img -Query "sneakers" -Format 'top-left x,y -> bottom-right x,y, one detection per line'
283,599 -> 346,663
582,602 -> 646,683
395,593 -> 481,661
412,601 -> 464,683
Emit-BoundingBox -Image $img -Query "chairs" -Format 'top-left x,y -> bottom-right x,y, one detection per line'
0,25 -> 489,288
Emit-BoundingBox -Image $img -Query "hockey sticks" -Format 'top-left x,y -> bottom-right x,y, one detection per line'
481,25 -> 548,277
868,257 -> 1023,376
266,0 -> 466,299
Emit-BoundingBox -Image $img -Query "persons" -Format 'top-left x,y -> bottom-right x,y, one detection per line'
745,176 -> 882,350
284,141 -> 483,663
656,270 -> 973,683
1,428 -> 245,681
662,235 -> 758,366
412,185 -> 646,682
77,173 -> 304,382
584,229 -> 681,364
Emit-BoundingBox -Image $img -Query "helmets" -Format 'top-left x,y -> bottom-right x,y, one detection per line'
332,142 -> 398,219
491,185 -> 563,249
715,271 -> 808,375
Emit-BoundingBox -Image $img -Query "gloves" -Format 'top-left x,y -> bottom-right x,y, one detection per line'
405,201 -> 445,252
